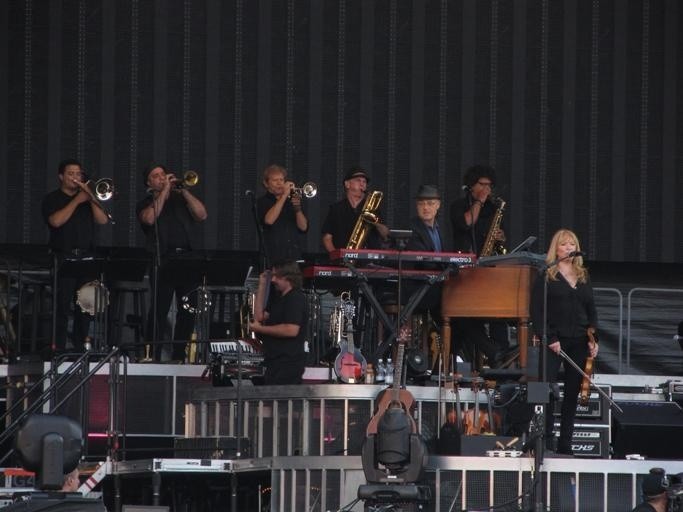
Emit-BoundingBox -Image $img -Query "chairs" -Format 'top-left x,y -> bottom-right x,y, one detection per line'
0,413 -> 108,511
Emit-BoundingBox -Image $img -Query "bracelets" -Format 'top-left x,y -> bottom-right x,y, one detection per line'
474,200 -> 485,208
293,204 -> 303,213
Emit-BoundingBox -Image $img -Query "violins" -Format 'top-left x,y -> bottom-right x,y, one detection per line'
580,326 -> 594,408
467,383 -> 482,438
449,383 -> 465,435
483,384 -> 504,436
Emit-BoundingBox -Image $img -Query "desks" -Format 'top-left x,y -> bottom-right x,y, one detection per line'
439,263 -> 536,375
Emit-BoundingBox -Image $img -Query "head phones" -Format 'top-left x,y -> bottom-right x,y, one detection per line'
650,468 -> 670,489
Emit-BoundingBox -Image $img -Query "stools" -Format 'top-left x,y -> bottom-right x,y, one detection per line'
380,297 -> 414,348
207,285 -> 249,337
109,281 -> 148,345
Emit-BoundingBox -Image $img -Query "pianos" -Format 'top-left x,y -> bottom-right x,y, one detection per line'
303,265 -> 448,281
209,342 -> 253,355
333,249 -> 477,270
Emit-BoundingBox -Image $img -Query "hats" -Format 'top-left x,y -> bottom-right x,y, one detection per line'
143,163 -> 166,187
345,168 -> 369,183
641,473 -> 671,496
412,183 -> 441,200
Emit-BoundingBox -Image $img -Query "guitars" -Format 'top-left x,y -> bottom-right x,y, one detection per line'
366,340 -> 417,437
334,300 -> 366,384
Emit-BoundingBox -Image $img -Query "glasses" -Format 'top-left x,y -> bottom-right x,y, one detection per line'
479,182 -> 493,186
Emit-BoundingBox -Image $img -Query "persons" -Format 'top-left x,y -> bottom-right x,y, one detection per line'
451,166 -> 508,368
255,164 -> 308,265
633,468 -> 674,512
396,184 -> 519,382
249,259 -> 307,384
135,163 -> 208,364
530,230 -> 600,456
42,161 -> 110,362
62,468 -> 81,492
321,169 -> 389,253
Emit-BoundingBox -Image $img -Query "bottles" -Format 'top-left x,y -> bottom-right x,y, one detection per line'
376,359 -> 385,380
385,358 -> 394,384
365,364 -> 374,383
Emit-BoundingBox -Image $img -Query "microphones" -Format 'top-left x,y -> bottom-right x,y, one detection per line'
674,334 -> 683,342
570,251 -> 586,258
245,189 -> 257,200
460,185 -> 476,202
146,187 -> 159,197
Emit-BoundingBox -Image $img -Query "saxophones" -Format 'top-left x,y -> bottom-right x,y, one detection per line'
481,196 -> 506,257
344,190 -> 384,248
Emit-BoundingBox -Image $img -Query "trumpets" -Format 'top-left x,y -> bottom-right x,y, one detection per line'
167,170 -> 199,188
286,181 -> 318,198
74,178 -> 116,202
